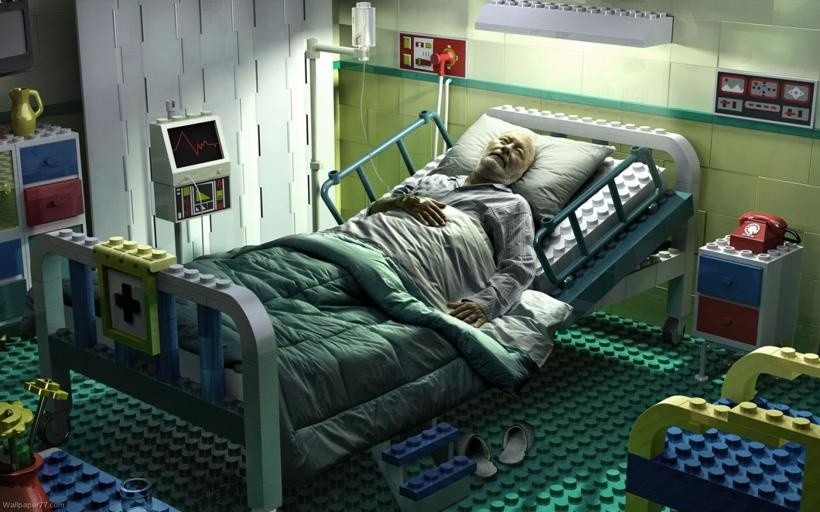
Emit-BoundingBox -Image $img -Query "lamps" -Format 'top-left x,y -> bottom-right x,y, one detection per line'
474,0 -> 674,47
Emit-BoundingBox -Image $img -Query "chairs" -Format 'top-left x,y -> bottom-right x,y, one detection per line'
625,345 -> 819,511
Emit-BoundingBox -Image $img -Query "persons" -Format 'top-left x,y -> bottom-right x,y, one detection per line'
366,128 -> 537,328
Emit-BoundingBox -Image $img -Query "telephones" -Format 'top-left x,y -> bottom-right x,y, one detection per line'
730,211 -> 787,253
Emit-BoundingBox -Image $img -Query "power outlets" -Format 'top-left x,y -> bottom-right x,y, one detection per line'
714,68 -> 818,127
399,33 -> 468,78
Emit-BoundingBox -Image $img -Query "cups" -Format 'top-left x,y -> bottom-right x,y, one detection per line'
119,476 -> 152,512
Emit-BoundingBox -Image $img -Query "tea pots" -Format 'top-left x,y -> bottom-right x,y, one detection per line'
9,86 -> 44,137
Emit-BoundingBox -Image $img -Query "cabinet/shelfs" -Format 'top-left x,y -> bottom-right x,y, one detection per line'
691,227 -> 804,382
0,121 -> 88,340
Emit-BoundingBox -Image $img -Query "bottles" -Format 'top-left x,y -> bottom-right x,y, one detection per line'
351,3 -> 377,63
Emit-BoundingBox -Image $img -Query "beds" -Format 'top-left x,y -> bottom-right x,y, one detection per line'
32,104 -> 704,512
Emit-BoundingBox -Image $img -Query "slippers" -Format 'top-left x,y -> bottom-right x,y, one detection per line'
454,430 -> 497,477
496,421 -> 535,463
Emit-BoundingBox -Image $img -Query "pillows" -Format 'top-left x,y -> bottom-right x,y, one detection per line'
426,112 -> 616,223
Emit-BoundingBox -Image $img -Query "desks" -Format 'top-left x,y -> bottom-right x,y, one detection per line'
34,447 -> 180,512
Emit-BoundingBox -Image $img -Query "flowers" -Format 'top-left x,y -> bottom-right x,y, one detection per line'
0,377 -> 69,469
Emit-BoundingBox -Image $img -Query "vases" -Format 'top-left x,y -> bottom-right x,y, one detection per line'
1,451 -> 51,512
